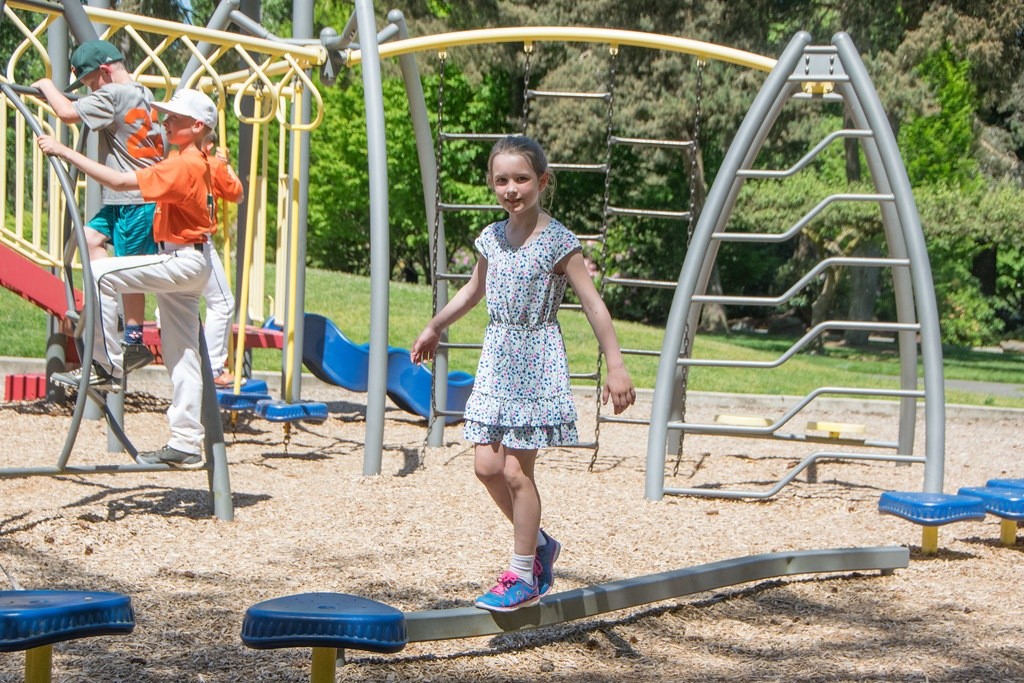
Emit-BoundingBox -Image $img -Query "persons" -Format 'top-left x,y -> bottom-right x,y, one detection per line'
38,88 -> 244,470
28,38 -> 250,386
409,137 -> 636,612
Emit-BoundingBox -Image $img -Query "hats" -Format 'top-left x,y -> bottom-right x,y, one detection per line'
62,39 -> 124,95
148,88 -> 218,130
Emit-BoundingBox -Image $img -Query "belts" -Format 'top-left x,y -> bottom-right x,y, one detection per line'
159,239 -> 203,251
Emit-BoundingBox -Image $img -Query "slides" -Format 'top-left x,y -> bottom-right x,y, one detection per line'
300,311 -> 479,426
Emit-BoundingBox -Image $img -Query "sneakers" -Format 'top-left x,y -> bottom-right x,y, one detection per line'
534,526 -> 561,599
475,570 -> 540,612
212,372 -> 248,388
49,361 -> 124,395
64,308 -> 83,321
133,443 -> 205,470
124,339 -> 153,378
